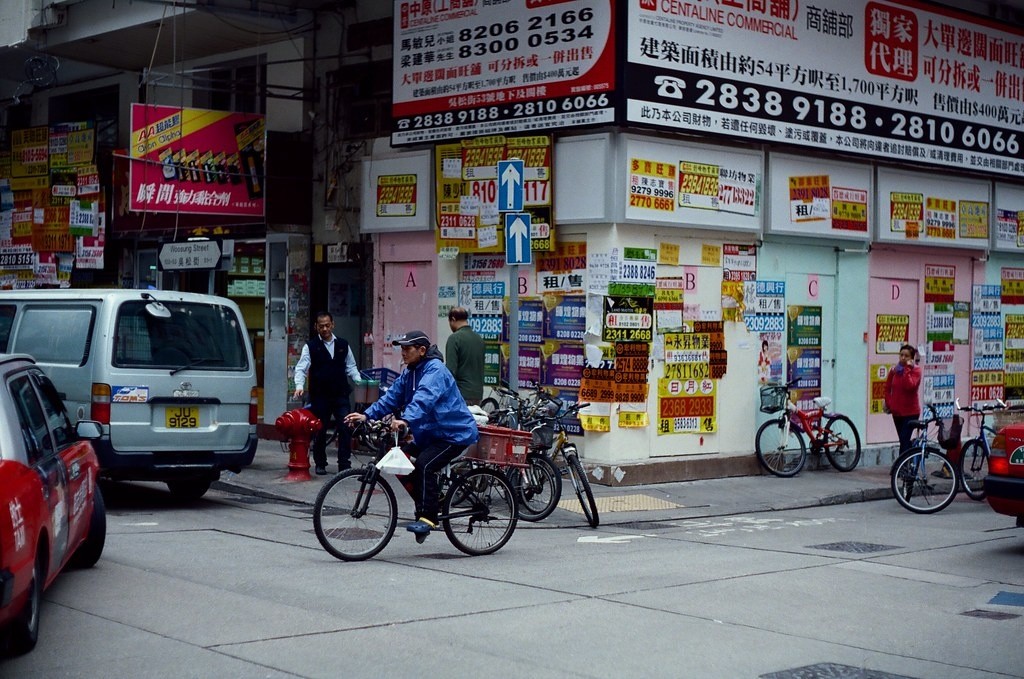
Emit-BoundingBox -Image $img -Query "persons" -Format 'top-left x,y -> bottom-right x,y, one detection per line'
344,331 -> 479,533
758,340 -> 770,382
292,312 -> 361,474
883,345 -> 921,453
445,307 -> 485,404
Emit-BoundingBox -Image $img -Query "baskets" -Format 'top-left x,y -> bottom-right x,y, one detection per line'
939,416 -> 965,450
526,421 -> 554,450
536,395 -> 563,417
760,384 -> 787,414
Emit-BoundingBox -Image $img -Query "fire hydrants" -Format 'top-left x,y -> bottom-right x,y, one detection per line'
274,410 -> 322,481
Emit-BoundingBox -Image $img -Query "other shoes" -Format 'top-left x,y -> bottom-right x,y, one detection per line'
338,462 -> 351,472
315,463 -> 327,475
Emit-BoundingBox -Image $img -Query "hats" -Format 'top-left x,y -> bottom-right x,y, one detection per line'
392,330 -> 431,348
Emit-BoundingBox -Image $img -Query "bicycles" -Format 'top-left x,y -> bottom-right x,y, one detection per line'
400,381 -> 601,530
755,376 -> 862,479
313,418 -> 518,564
889,404 -> 958,516
955,396 -> 1007,503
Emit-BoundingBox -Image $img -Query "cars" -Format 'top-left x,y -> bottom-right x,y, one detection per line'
0,351 -> 107,657
984,422 -> 1024,527
983,420 -> 1024,528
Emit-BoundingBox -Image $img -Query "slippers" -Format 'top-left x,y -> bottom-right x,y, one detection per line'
406,516 -> 440,533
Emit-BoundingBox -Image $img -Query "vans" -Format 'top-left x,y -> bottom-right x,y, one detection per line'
1,288 -> 259,499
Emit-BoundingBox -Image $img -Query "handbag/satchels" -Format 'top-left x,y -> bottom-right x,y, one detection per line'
375,431 -> 415,475
882,400 -> 891,414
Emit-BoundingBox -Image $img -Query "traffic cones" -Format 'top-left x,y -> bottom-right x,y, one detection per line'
931,414 -> 973,480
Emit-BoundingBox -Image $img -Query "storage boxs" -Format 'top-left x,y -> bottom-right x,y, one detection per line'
353,378 -> 380,404
459,425 -> 533,465
359,367 -> 402,399
